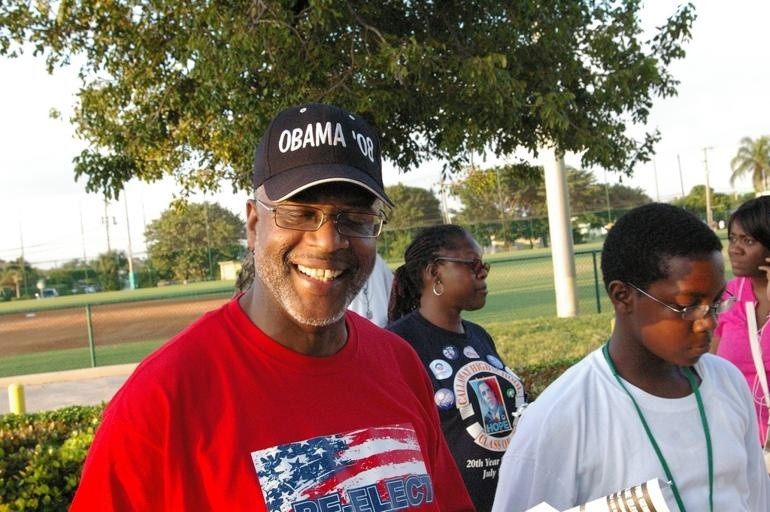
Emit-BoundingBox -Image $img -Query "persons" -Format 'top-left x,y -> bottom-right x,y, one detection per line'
71,103 -> 483,512
382,226 -> 528,512
476,378 -> 510,434
488,201 -> 770,511
710,193 -> 770,473
349,253 -> 397,329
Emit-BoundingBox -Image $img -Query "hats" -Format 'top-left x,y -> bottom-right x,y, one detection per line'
252,101 -> 397,211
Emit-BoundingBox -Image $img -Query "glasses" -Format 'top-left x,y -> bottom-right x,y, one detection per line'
625,279 -> 737,323
430,256 -> 491,276
254,197 -> 385,239
